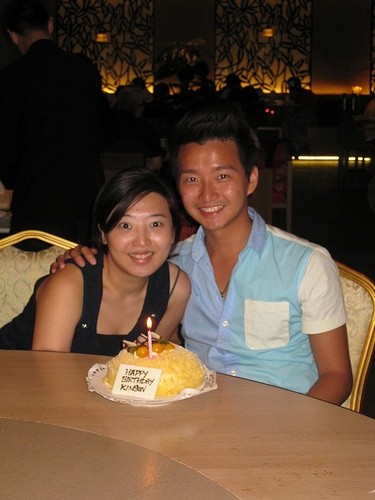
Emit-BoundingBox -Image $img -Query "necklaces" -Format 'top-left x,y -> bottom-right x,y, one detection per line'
217,279 -> 230,299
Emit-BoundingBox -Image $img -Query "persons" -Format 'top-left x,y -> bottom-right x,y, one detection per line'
108,71 -> 318,199
0,168 -> 191,357
1,0 -> 110,252
50,111 -> 354,406
362,83 -> 375,146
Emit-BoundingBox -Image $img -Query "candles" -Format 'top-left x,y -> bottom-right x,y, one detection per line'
146,317 -> 153,360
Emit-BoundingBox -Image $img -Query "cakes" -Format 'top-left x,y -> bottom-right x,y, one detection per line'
102,331 -> 205,398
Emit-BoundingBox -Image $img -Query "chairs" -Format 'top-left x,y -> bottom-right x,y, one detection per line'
0,230 -> 78,328
330,261 -> 375,413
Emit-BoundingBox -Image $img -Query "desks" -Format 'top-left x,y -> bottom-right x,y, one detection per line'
0,350 -> 375,500
